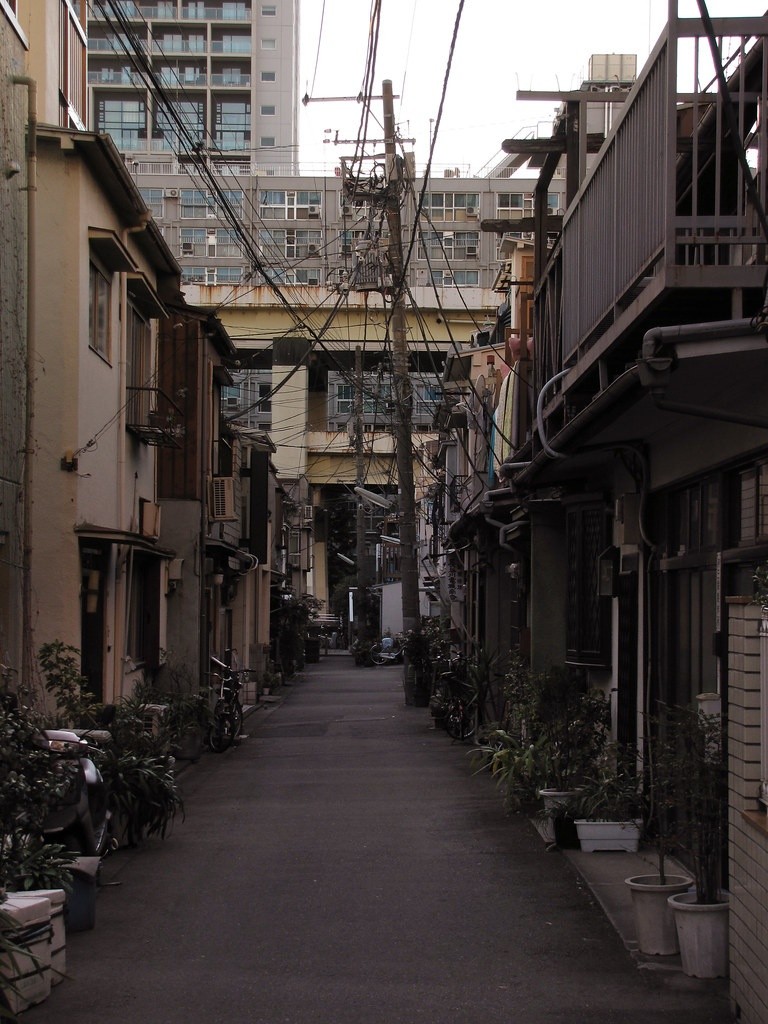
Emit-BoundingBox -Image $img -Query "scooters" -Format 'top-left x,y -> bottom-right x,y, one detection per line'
0,692 -> 115,889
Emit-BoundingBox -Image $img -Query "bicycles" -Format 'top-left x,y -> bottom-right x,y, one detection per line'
369,632 -> 410,666
203,655 -> 257,754
431,650 -> 483,745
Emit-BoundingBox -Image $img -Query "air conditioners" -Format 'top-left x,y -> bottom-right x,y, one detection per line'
182,242 -> 194,252
466,206 -> 478,216
308,243 -> 319,253
466,246 -> 479,255
341,244 -> 352,254
165,189 -> 178,198
308,205 -> 319,215
307,278 -> 319,287
547,207 -> 557,215
341,205 -> 353,216
137,704 -> 171,753
212,476 -> 241,522
304,505 -> 316,523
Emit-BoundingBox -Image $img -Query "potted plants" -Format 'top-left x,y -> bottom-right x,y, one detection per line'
537,685 -> 612,841
167,687 -> 219,760
653,700 -> 729,979
87,749 -> 185,848
612,709 -> 695,956
261,673 -> 280,695
304,635 -> 321,663
399,624 -> 442,708
534,797 -> 583,849
571,775 -> 652,853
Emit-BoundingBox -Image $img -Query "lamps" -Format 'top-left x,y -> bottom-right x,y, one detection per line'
81,569 -> 102,614
169,559 -> 183,589
